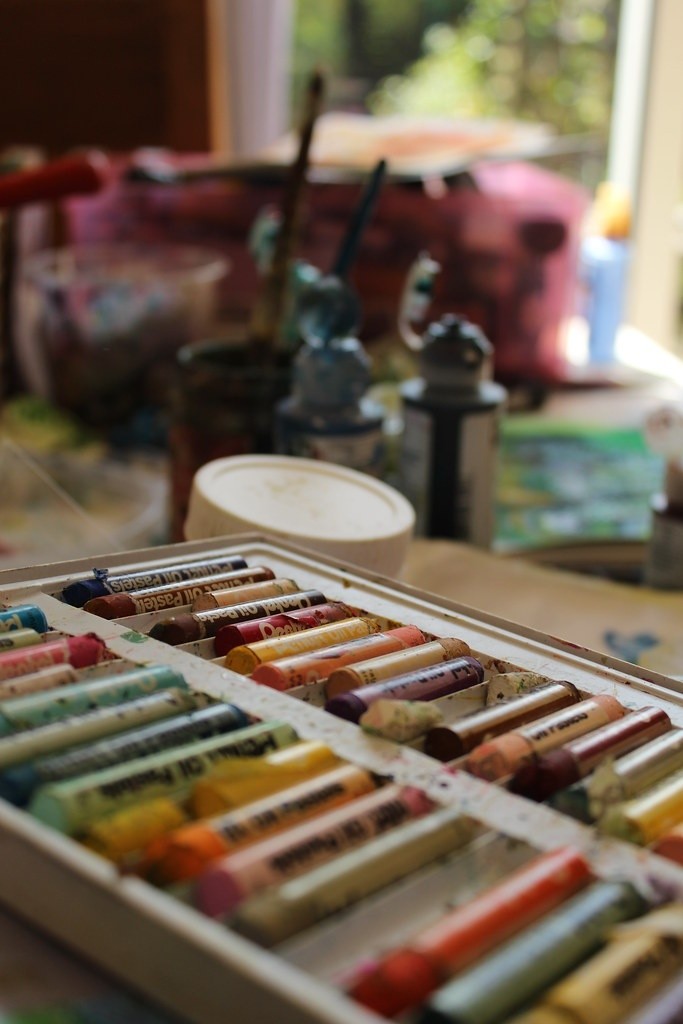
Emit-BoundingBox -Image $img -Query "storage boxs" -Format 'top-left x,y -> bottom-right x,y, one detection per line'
492,421 -> 663,504
490,508 -> 656,557
0,532 -> 683,1024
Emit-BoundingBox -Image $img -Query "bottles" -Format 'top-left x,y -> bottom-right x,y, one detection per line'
5,105 -> 682,593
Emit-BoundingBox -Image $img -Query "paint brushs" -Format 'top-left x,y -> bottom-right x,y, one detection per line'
263,68 -> 326,340
328,156 -> 390,278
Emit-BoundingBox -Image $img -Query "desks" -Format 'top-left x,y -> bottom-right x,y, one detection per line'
0,540 -> 683,1024
